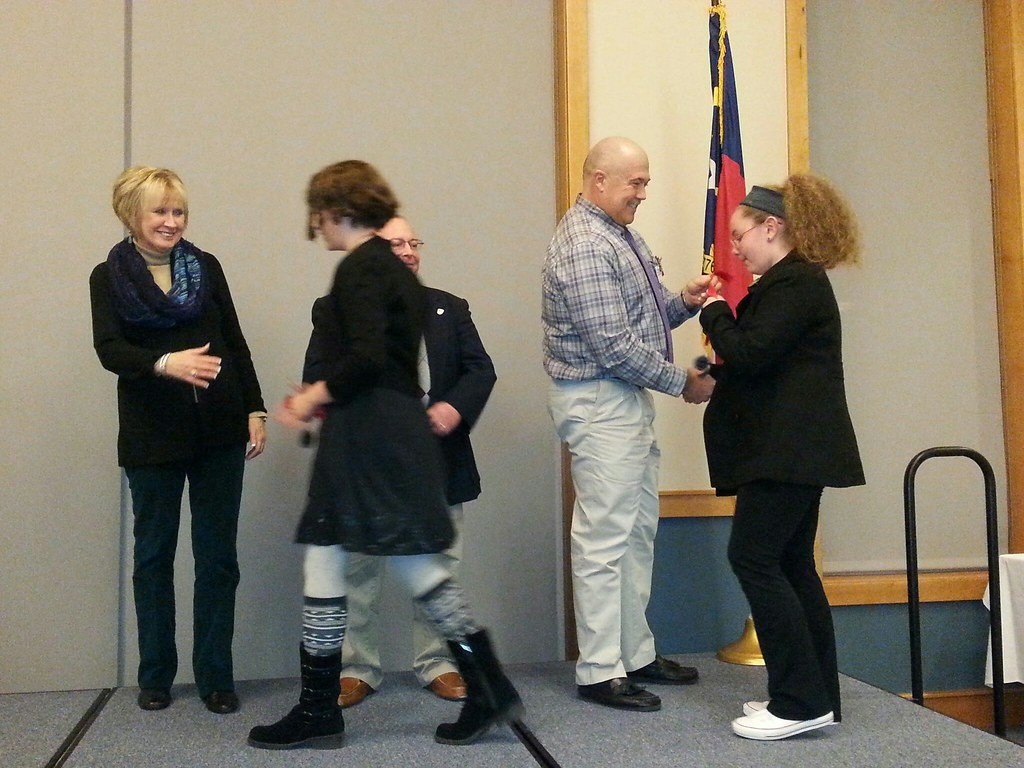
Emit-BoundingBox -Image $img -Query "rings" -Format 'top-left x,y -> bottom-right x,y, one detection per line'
191,369 -> 198,376
440,422 -> 446,428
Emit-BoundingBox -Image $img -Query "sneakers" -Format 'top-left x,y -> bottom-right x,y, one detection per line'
730,700 -> 840,740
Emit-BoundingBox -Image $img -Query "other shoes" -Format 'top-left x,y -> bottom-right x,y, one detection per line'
578,676 -> 661,713
138,690 -> 169,711
198,688 -> 237,715
626,655 -> 699,684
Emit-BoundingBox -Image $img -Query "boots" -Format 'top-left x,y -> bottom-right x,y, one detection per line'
434,626 -> 525,745
247,641 -> 345,749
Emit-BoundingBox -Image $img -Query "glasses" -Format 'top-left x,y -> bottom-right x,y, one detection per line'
389,238 -> 425,253
728,221 -> 764,247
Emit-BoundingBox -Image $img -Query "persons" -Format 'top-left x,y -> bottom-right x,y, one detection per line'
247,160 -> 524,750
684,174 -> 865,742
90,166 -> 267,714
335,214 -> 499,708
542,137 -> 720,710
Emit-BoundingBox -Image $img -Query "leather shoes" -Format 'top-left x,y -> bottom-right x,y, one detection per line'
426,672 -> 467,701
338,677 -> 372,707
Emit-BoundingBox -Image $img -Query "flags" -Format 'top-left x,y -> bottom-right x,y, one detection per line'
702,8 -> 752,363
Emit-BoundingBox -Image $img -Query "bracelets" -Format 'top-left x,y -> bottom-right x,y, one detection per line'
159,353 -> 171,376
